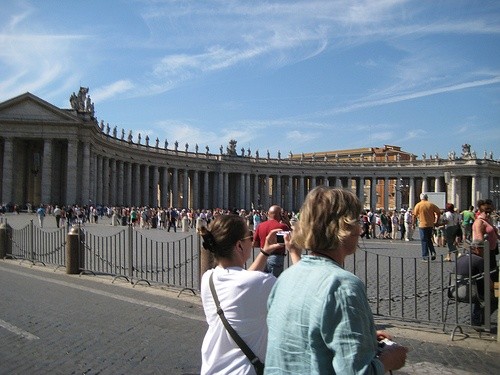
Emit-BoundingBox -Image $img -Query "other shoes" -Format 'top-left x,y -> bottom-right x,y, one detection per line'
422,259 -> 428,263
444,257 -> 451,261
458,252 -> 461,258
431,255 -> 436,261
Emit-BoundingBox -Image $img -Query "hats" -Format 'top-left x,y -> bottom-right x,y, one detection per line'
399,209 -> 406,212
408,208 -> 412,212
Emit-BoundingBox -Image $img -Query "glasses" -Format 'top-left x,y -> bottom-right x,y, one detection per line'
240,231 -> 253,242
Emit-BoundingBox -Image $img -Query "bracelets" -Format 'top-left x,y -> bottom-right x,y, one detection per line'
260,248 -> 270,256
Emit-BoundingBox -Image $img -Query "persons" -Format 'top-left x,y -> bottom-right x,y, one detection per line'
437,203 -> 462,261
54,205 -> 62,228
200,214 -> 301,375
360,206 -> 500,247
252,206 -> 290,277
61,205 -> 300,228
453,199 -> 500,328
414,193 -> 440,263
253,210 -> 261,230
166,207 -> 176,232
36,206 -> 46,227
263,186 -> 409,375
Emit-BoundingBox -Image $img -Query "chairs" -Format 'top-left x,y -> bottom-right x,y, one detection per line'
443,272 -> 486,334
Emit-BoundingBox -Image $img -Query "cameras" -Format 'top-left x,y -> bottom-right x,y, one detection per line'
276,232 -> 289,245
377,338 -> 397,353
